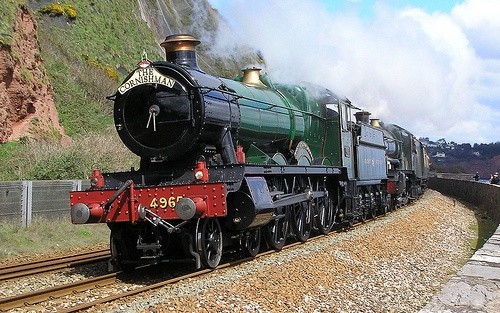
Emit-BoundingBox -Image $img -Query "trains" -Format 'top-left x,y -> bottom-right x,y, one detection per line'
71,34 -> 433,275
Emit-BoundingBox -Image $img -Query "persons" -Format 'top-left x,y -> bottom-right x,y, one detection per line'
473,171 -> 480,180
490,171 -> 500,184
